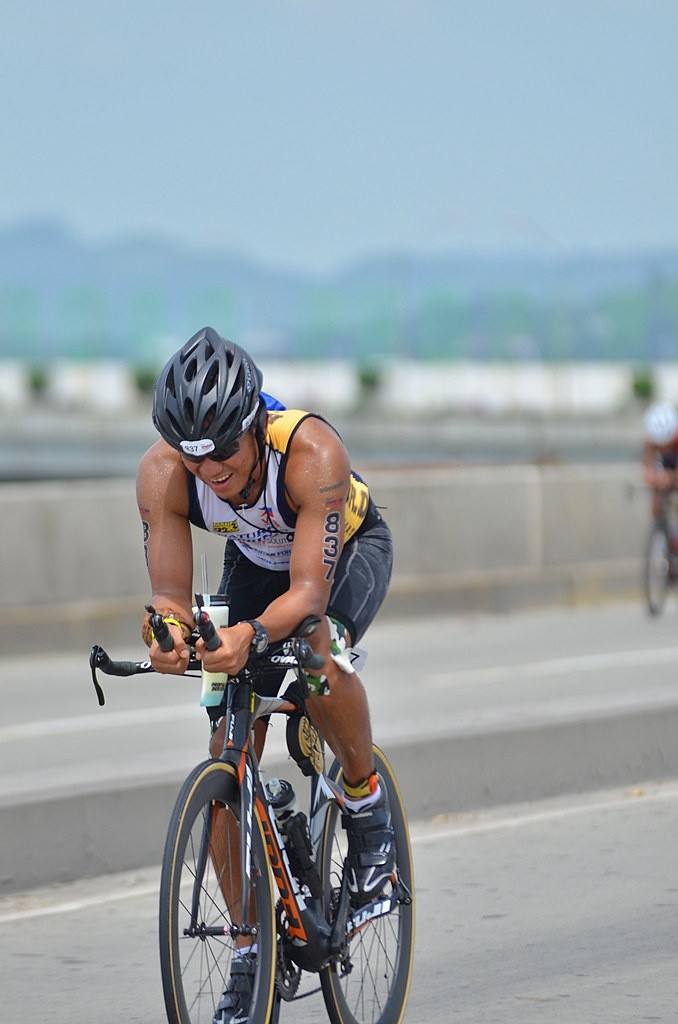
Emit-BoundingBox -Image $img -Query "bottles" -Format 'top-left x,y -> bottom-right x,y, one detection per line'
265,777 -> 316,865
191,593 -> 228,706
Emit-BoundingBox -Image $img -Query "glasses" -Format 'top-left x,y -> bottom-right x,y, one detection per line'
182,398 -> 259,464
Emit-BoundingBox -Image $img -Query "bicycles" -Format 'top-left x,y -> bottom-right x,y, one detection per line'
643,460 -> 678,614
89,593 -> 415,1024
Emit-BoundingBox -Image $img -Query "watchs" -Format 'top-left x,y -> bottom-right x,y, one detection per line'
243,619 -> 269,657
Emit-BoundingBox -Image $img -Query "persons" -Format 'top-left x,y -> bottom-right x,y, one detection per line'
135,326 -> 397,1023
640,402 -> 678,583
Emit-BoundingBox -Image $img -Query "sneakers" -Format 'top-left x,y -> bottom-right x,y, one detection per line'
341,772 -> 395,903
212,952 -> 257,1024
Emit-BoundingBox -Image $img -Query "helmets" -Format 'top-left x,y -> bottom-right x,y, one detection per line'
152,326 -> 263,457
644,402 -> 677,445
